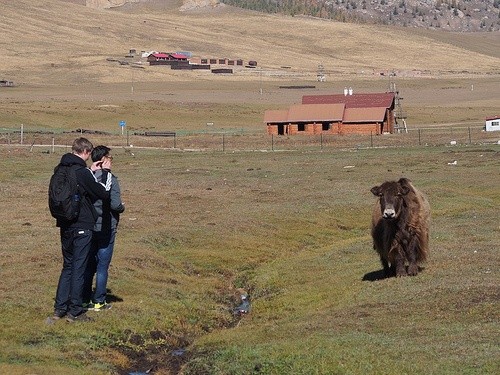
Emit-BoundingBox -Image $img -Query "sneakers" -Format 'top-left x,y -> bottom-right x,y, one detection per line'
52,308 -> 67,320
82,303 -> 94,310
66,313 -> 95,324
93,301 -> 112,312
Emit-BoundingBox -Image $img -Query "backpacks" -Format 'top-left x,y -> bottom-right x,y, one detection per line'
48,164 -> 87,223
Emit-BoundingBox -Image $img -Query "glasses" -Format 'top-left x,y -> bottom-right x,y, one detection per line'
105,155 -> 113,159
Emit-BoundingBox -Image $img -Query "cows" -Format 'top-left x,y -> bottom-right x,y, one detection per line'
370,177 -> 431,278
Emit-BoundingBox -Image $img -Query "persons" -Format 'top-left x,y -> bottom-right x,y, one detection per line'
82,145 -> 125,312
53,137 -> 113,325
